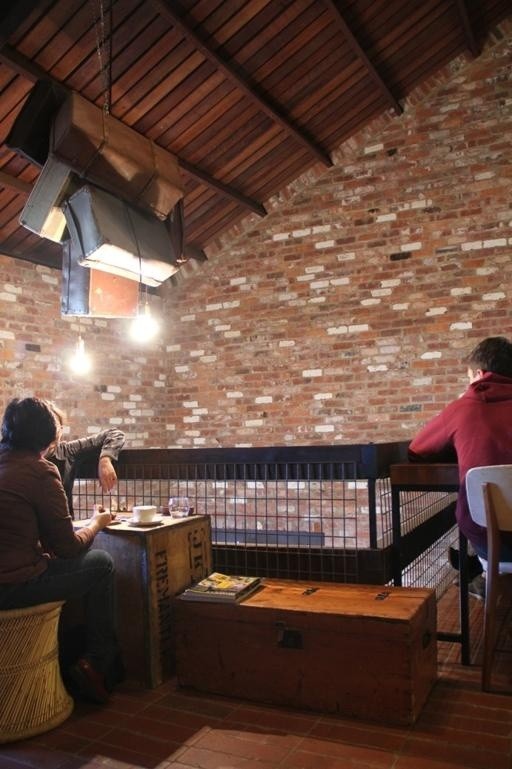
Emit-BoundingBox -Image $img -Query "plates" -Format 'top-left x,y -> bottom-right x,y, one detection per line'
127,518 -> 163,525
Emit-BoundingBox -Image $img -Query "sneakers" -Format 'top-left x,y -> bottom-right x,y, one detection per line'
76,658 -> 110,703
462,575 -> 485,600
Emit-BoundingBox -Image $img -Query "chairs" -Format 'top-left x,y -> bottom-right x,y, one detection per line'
464,464 -> 512,693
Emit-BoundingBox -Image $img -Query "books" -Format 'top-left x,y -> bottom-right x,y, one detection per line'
180,571 -> 262,604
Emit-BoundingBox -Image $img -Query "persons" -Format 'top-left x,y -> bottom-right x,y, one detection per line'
0,395 -> 117,705
43,398 -> 127,520
407,334 -> 512,586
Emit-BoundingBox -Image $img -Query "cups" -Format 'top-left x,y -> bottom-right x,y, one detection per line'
133,505 -> 157,521
167,497 -> 190,517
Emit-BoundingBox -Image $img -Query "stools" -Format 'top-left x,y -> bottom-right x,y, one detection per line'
0,596 -> 76,747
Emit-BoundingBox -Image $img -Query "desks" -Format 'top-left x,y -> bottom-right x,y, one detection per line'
388,460 -> 472,667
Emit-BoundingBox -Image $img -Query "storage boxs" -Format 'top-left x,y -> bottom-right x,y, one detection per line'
71,512 -> 215,690
173,575 -> 438,731
